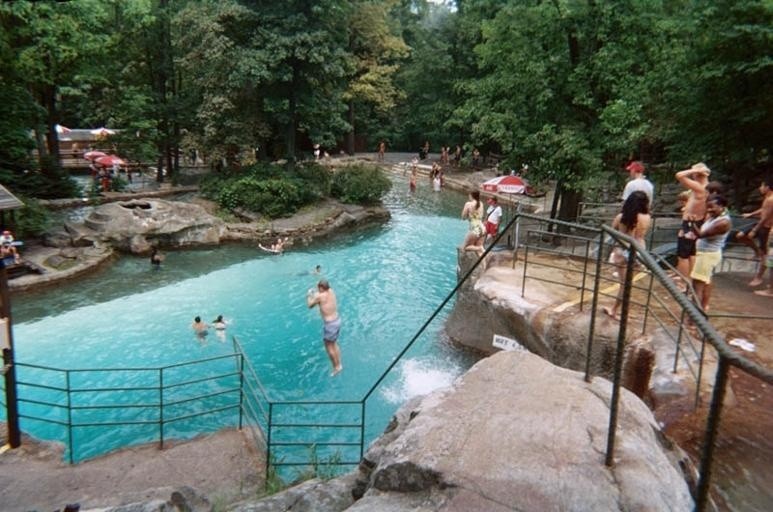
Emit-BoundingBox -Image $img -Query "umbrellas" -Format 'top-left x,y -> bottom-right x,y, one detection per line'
484,172 -> 543,222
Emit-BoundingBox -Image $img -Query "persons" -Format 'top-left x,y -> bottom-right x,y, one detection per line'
0,231 -> 18,265
192,316 -> 210,337
314,141 -> 528,193
307,279 -> 343,376
182,145 -> 196,164
212,315 -> 226,330
72,140 -> 132,192
461,189 -> 487,271
314,265 -> 321,275
151,248 -> 165,264
603,161 -> 773,338
275,238 -> 286,254
484,195 -> 503,245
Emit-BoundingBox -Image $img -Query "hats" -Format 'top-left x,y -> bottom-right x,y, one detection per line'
625,161 -> 645,172
